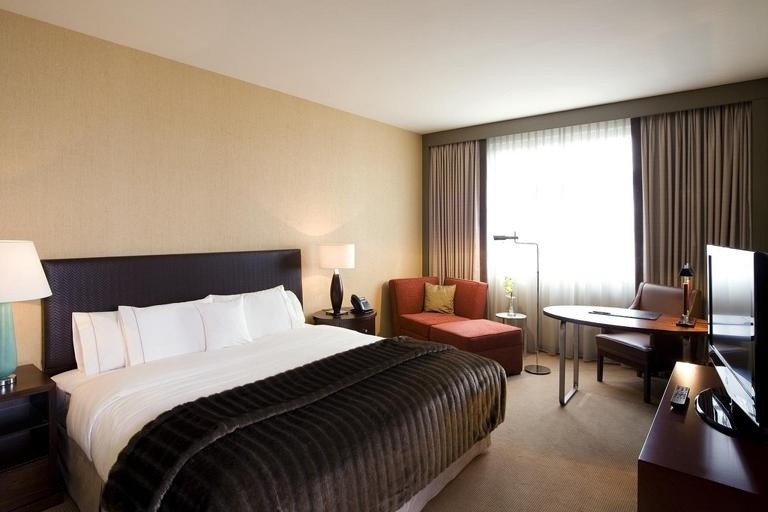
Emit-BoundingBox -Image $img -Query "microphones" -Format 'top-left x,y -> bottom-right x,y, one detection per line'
493,234 -> 519,241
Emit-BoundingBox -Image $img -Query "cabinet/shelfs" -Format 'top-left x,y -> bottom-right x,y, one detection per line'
637,358 -> 768,512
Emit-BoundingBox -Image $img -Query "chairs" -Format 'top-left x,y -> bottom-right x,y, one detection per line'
594,282 -> 702,405
389,272 -> 523,375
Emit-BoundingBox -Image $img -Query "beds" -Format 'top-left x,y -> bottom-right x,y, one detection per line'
40,247 -> 506,511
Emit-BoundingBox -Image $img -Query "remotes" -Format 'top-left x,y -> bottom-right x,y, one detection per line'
669,384 -> 690,406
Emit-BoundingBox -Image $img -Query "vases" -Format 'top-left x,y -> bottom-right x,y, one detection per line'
508,298 -> 515,317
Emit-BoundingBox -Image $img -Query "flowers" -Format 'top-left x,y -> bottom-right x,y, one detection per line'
502,276 -> 516,298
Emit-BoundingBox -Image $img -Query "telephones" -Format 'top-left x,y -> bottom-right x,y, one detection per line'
351,294 -> 373,311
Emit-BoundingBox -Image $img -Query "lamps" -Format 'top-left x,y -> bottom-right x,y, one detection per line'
493,232 -> 551,375
676,262 -> 696,329
317,244 -> 355,312
0,239 -> 53,386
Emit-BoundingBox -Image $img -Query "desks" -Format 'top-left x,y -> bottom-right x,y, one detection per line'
542,304 -> 708,408
495,313 -> 527,329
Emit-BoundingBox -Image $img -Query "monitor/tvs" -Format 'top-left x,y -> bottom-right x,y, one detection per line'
694,243 -> 768,439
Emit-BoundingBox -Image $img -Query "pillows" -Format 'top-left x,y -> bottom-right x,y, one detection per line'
69,283 -> 309,372
422,282 -> 457,315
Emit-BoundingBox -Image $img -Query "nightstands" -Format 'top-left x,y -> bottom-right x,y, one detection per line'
311,306 -> 378,335
0,362 -> 57,512
